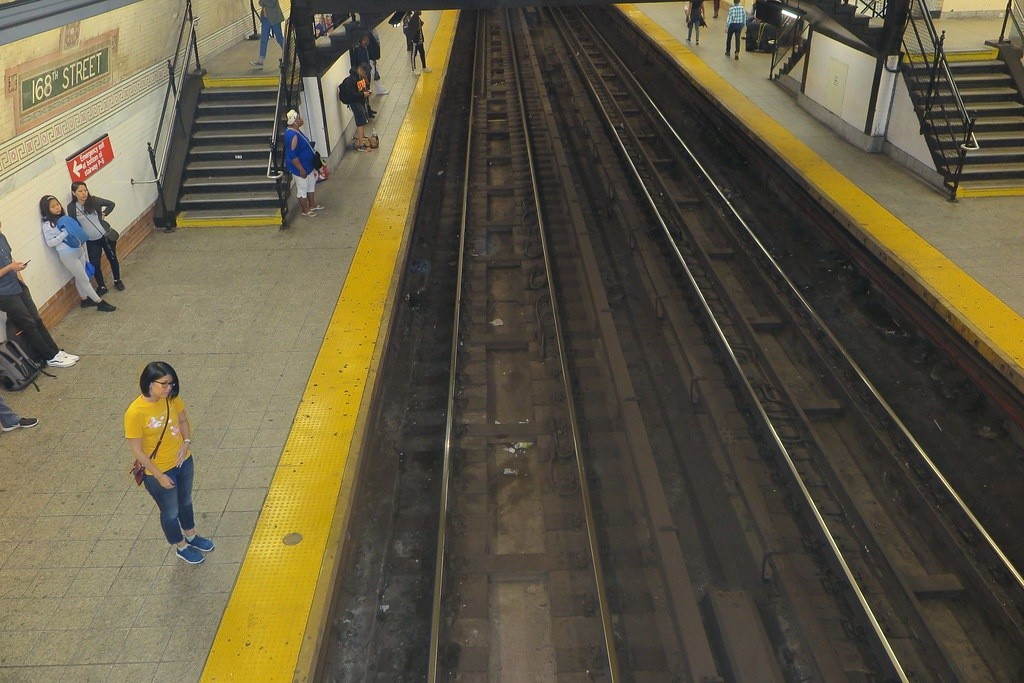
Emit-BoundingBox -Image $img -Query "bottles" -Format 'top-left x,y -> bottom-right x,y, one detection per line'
371,132 -> 378,147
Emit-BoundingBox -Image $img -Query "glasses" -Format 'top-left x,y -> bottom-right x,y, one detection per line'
152,379 -> 175,388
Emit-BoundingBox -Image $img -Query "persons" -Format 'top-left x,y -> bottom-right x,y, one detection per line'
0,396 -> 39,431
124,361 -> 215,564
283,109 -> 324,218
403,9 -> 431,75
712,0 -> 720,18
354,36 -> 389,94
725,0 -> 747,60
0,223 -> 79,367
40,195 -> 116,312
686,0 -> 704,45
249,0 -> 286,68
66,182 -> 125,294
345,63 -> 373,152
313,15 -> 333,37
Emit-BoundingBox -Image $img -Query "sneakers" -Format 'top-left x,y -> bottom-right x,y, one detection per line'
46,351 -> 78,368
185,535 -> 216,551
1,417 -> 39,431
176,545 -> 205,564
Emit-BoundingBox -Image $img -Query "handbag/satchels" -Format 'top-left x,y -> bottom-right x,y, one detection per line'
104,229 -> 119,245
353,134 -> 379,148
129,459 -> 146,486
312,151 -> 322,170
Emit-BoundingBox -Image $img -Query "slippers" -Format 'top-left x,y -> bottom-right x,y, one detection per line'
302,211 -> 318,217
310,204 -> 325,210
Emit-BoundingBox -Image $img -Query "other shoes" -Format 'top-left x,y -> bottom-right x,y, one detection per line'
735,51 -> 739,60
97,285 -> 108,297
375,88 -> 390,95
81,296 -> 98,308
367,110 -> 378,118
249,61 -> 263,69
97,300 -> 116,312
114,279 -> 125,290
412,67 -> 432,75
725,52 -> 730,56
358,144 -> 372,152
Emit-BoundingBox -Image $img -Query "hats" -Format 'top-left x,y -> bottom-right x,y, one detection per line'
287,110 -> 298,125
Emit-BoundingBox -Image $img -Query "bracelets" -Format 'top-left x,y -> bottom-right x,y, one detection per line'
183,439 -> 191,443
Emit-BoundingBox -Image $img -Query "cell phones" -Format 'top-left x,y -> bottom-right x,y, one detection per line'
22,260 -> 31,266
369,92 -> 372,94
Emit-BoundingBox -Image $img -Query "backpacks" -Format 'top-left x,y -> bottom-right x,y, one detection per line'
0,339 -> 39,391
53,216 -> 89,248
338,77 -> 358,104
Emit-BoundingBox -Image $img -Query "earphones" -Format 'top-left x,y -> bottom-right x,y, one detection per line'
295,122 -> 296,124
